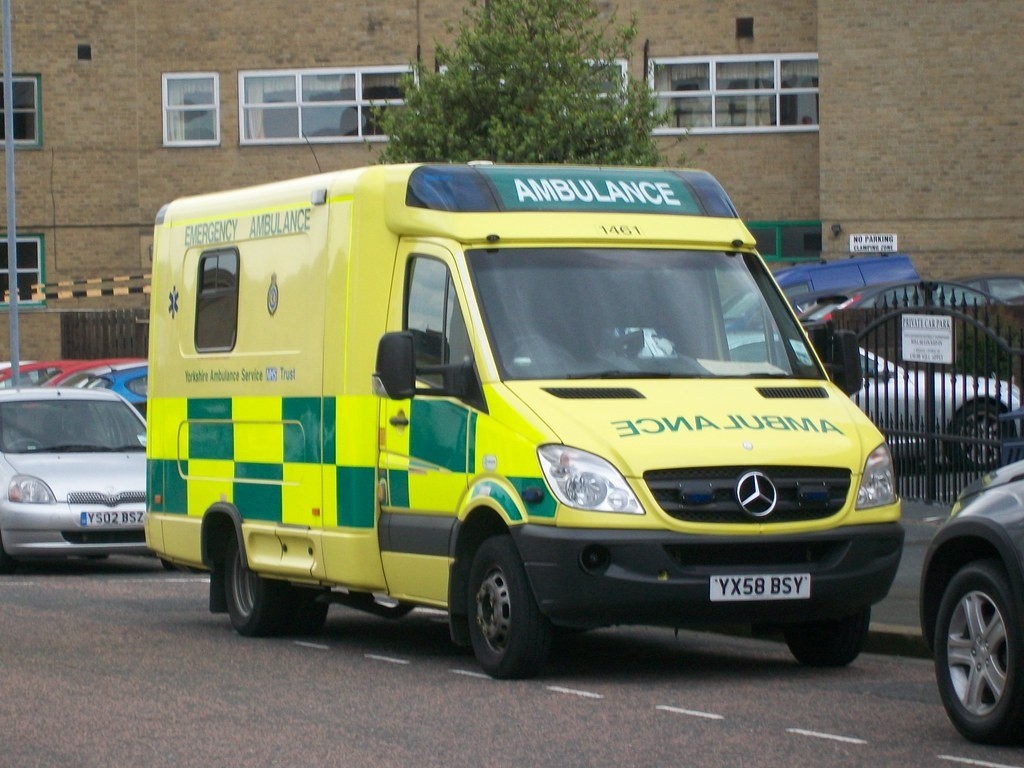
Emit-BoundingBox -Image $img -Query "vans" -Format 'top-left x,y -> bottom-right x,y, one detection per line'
720,254 -> 918,338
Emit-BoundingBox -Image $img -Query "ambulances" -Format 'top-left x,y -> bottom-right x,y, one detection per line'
145,160 -> 907,679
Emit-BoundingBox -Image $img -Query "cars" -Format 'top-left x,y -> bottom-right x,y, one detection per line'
788,268 -> 1023,345
0,358 -> 151,414
917,457 -> 1024,748
0,388 -> 168,573
730,331 -> 1024,474
